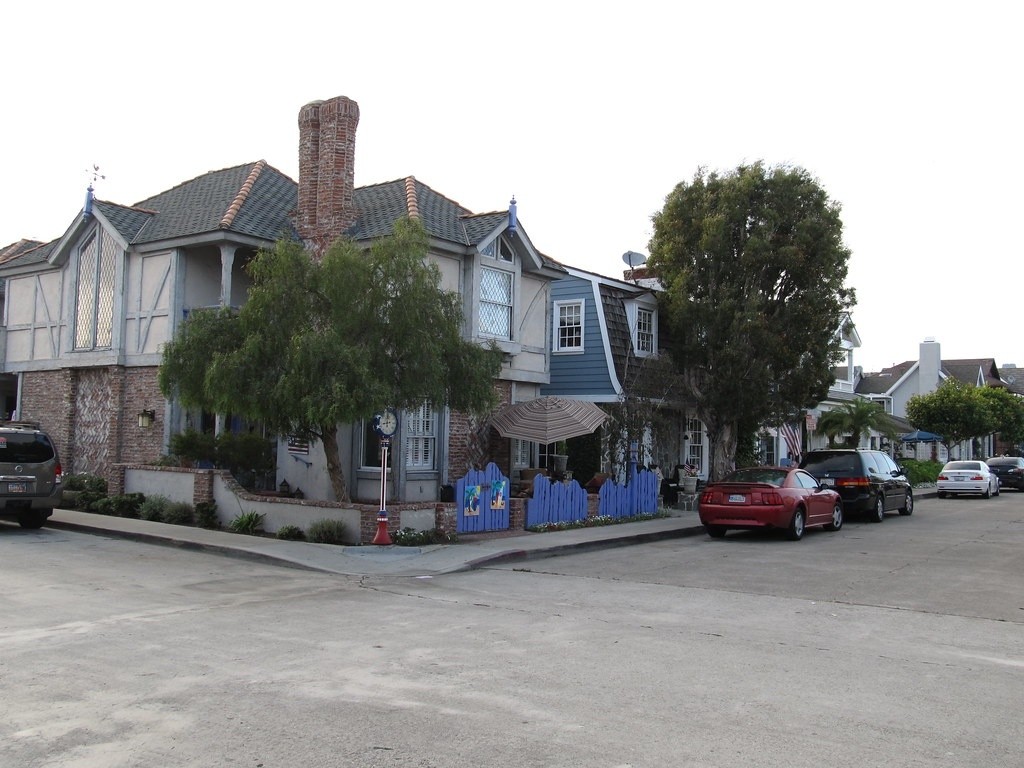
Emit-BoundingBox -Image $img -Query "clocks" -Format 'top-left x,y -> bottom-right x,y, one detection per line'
373,408 -> 399,440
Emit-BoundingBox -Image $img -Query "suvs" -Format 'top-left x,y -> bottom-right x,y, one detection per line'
799,448 -> 914,523
0,420 -> 65,531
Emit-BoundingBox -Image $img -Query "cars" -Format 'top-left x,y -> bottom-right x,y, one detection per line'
936,460 -> 1000,500
697,466 -> 843,541
985,456 -> 1024,493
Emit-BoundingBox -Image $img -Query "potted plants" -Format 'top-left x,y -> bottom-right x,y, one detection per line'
552,440 -> 569,471
682,472 -> 698,493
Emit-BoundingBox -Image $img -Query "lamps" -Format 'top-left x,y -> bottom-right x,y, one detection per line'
137,409 -> 155,427
